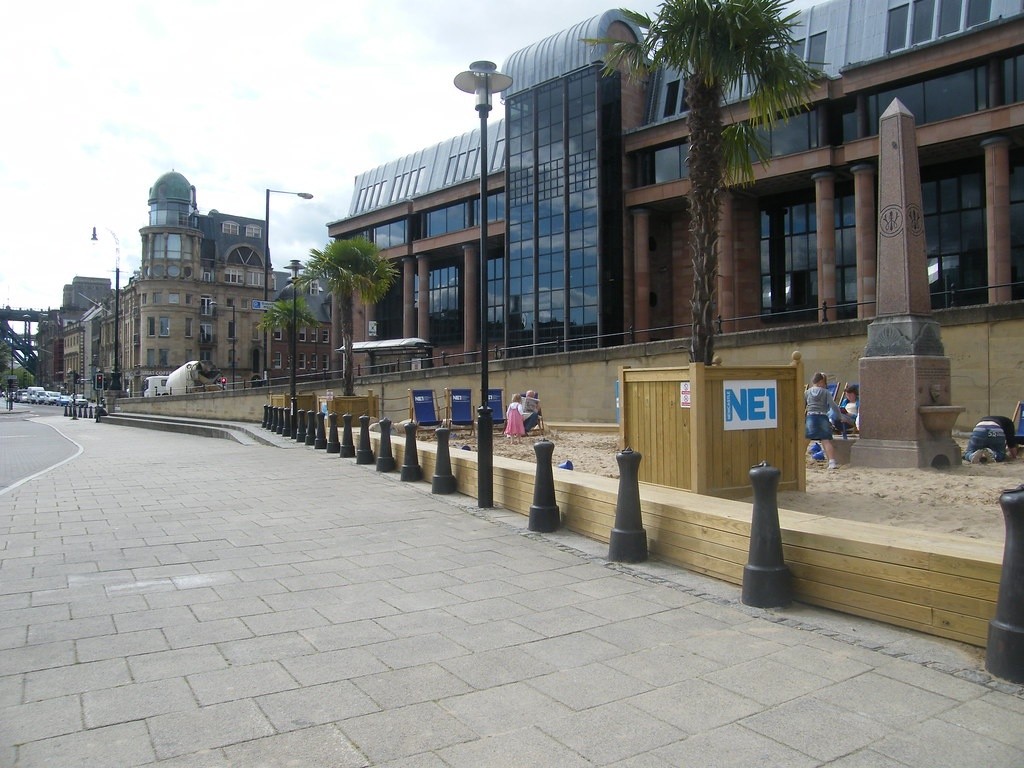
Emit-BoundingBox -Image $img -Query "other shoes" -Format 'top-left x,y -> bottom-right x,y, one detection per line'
983,448 -> 996,463
971,449 -> 984,464
829,459 -> 837,469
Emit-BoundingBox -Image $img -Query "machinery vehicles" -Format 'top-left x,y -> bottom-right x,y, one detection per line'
143,359 -> 226,397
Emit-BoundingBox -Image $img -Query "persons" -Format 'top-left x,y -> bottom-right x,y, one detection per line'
800,372 -> 858,469
961,415 -> 1018,463
95,405 -> 108,423
505,389 -> 541,445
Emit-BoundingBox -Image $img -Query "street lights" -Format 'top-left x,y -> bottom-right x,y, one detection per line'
283,258 -> 306,441
91,225 -> 121,390
207,301 -> 235,390
454,59 -> 515,405
262,189 -> 314,381
21,313 -> 32,348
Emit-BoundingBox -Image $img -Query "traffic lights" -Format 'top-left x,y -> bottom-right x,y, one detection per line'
96,372 -> 103,391
220,376 -> 227,386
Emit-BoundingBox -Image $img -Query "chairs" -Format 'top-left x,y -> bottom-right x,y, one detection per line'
801,381 -> 860,439
1004,400 -> 1024,447
404,386 -> 545,439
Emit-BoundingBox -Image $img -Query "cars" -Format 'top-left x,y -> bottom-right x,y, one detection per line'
69,393 -> 88,408
55,395 -> 71,406
2,386 -> 61,406
